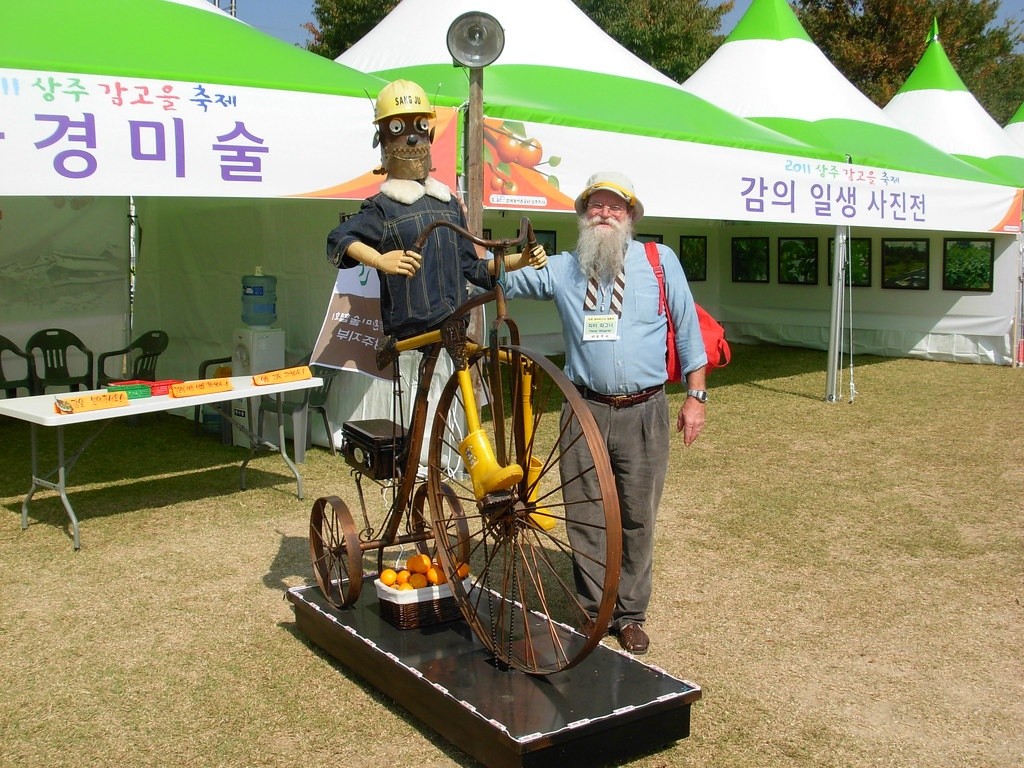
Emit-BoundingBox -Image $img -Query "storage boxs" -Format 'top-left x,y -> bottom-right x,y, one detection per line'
482,229 -> 492,252
342,420 -> 410,480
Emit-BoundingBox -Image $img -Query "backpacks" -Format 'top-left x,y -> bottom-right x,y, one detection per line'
644,241 -> 731,384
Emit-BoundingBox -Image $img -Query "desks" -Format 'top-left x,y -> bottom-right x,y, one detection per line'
0,376 -> 324,549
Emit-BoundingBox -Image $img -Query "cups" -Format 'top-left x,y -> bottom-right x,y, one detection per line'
254,265 -> 264,276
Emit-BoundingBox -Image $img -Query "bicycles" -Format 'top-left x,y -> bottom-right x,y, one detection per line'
308,218 -> 624,676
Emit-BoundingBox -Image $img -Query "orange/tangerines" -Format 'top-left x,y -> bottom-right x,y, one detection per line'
214,366 -> 233,378
380,554 -> 471,591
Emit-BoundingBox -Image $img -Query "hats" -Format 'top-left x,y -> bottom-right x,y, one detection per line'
574,172 -> 644,222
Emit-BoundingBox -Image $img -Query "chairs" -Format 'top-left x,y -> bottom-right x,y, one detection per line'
0,336 -> 42,398
97,329 -> 168,388
27,328 -> 94,395
194,356 -> 233,446
249,349 -> 339,456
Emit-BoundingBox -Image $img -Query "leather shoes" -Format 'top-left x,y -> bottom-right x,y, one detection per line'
618,623 -> 650,655
587,622 -> 609,638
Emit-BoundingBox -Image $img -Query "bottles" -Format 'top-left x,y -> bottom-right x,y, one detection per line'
241,276 -> 277,326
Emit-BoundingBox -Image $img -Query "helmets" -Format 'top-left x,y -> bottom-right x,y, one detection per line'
372,79 -> 436,123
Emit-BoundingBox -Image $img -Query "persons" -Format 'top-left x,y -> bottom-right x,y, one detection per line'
326,79 -> 558,532
458,170 -> 709,654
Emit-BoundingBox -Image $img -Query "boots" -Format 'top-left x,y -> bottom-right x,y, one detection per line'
458,429 -> 524,504
527,456 -> 556,531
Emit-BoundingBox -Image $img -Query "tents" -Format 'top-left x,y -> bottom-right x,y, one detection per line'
0,0 -> 1024,483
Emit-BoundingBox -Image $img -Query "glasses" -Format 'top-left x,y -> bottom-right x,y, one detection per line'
587,202 -> 628,211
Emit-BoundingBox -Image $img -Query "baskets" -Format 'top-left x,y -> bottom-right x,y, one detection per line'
146,380 -> 184,396
111,379 -> 149,385
104,384 -> 151,399
374,567 -> 472,630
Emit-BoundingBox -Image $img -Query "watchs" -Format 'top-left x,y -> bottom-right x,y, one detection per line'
687,390 -> 708,402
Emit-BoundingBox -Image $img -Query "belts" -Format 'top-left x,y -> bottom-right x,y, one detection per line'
573,383 -> 663,408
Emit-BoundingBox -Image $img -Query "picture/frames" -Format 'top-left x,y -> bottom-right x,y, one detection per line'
828,237 -> 872,287
517,229 -> 557,257
680,235 -> 707,282
942,237 -> 993,291
634,233 -> 664,244
731,237 -> 769,283
880,237 -> 932,290
777,237 -> 819,285
